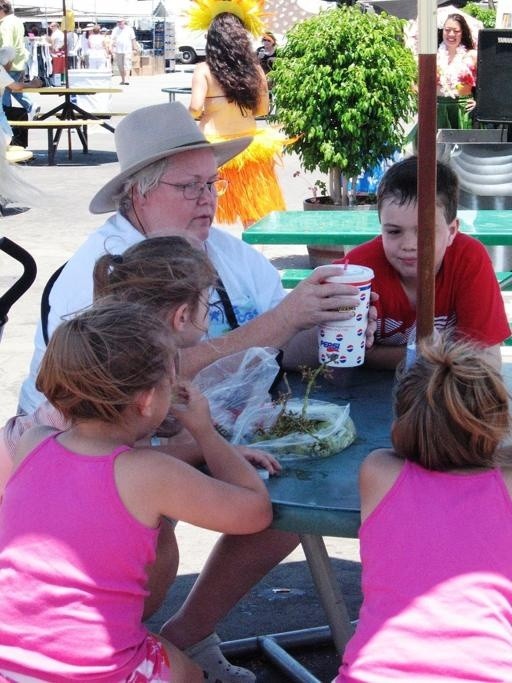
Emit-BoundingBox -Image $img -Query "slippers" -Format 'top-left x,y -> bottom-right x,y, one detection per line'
179,630 -> 256,683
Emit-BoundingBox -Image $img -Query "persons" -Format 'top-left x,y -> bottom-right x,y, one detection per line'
404,13 -> 477,154
17,101 -> 380,682
0,0 -> 137,205
256,32 -> 277,119
1,302 -> 273,682
330,327 -> 512,683
0,235 -> 216,483
331,156 -> 512,377
188,0 -> 284,223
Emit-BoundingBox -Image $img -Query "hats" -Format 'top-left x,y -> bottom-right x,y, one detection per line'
89,101 -> 254,214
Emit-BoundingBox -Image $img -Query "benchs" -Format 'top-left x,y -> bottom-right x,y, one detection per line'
36,111 -> 111,154
7,121 -> 104,166
281,268 -> 512,292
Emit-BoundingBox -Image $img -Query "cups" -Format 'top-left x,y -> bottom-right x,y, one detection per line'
55,74 -> 61,85
316,265 -> 375,369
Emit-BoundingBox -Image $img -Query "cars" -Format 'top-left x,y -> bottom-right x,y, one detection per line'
174,25 -> 288,62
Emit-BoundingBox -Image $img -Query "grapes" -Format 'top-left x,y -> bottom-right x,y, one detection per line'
249,417 -> 355,458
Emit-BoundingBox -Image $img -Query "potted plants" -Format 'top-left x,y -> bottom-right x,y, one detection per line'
267,3 -> 418,270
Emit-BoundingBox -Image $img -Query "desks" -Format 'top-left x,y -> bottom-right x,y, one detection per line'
11,88 -> 122,160
242,210 -> 512,247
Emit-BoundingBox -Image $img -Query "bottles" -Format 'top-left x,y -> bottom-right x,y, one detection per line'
407,327 -> 442,371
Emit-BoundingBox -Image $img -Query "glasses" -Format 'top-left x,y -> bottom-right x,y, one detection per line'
158,179 -> 228,201
